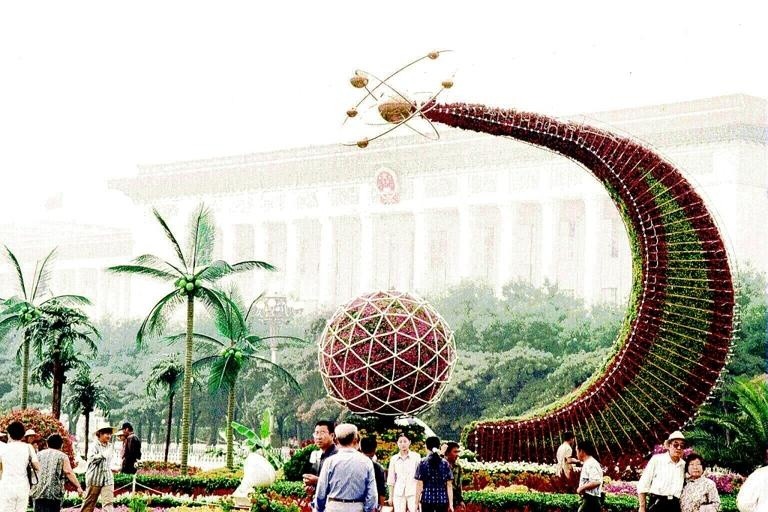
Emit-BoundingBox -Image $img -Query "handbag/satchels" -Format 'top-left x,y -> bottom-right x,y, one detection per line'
27,462 -> 38,485
64,481 -> 77,490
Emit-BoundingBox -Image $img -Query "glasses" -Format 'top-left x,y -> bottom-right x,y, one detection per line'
674,444 -> 685,449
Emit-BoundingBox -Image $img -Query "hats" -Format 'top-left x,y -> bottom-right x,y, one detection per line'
666,430 -> 685,443
24,429 -> 37,436
93,423 -> 133,435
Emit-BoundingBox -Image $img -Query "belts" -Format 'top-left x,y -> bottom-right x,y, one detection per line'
329,498 -> 362,502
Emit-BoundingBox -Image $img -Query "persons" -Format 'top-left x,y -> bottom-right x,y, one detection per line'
0,420 -> 142,512
734,437 -> 768,512
680,453 -> 721,512
301,419 -> 466,512
636,431 -> 689,512
574,441 -> 603,512
556,432 -> 581,480
